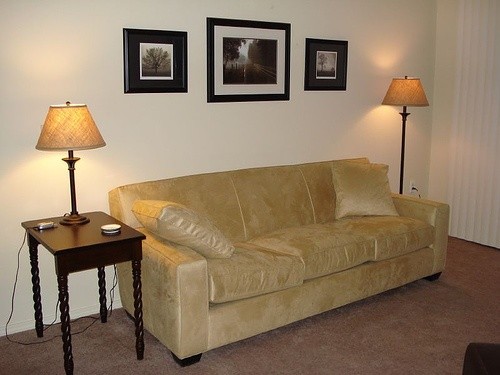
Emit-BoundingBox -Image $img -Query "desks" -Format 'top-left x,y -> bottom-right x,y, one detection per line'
21,213 -> 146,375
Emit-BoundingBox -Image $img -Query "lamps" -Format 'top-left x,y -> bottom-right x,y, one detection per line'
35,101 -> 106,226
382,76 -> 429,195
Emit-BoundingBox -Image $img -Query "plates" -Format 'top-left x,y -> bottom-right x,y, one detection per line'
100,224 -> 121,234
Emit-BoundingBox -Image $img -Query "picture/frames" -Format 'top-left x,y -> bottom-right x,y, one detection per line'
304,37 -> 349,91
206,17 -> 290,103
122,27 -> 188,94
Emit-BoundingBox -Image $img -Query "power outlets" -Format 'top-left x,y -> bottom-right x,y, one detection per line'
409,179 -> 416,193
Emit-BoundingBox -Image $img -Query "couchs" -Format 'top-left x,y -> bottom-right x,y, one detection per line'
108,157 -> 450,366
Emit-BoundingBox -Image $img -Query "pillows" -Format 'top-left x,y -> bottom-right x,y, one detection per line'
133,198 -> 234,259
331,161 -> 398,219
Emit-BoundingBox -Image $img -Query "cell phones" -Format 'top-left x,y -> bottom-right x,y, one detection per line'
38,221 -> 57,229
